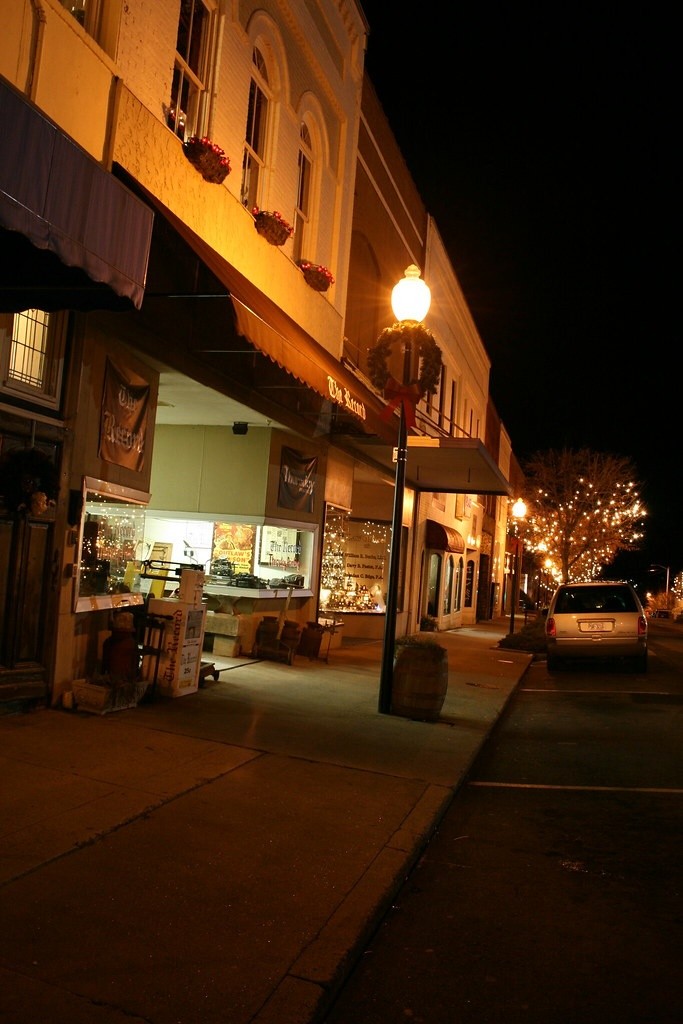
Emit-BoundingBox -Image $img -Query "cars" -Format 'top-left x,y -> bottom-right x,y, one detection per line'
540,581 -> 650,674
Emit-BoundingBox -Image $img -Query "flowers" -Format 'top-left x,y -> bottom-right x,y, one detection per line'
252,206 -> 294,233
301,263 -> 334,284
190,136 -> 232,173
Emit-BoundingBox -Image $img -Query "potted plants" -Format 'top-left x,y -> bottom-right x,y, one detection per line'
71,674 -> 149,716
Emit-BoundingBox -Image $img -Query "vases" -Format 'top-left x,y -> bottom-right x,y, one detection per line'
256,218 -> 290,245
187,145 -> 230,184
305,271 -> 330,291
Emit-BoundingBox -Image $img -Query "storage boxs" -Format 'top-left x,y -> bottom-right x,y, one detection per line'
141,596 -> 208,699
178,569 -> 205,605
211,636 -> 241,658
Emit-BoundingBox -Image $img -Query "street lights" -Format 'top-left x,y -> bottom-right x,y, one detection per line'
650,563 -> 669,608
376,260 -> 434,719
505,497 -> 528,635
535,543 -> 547,609
